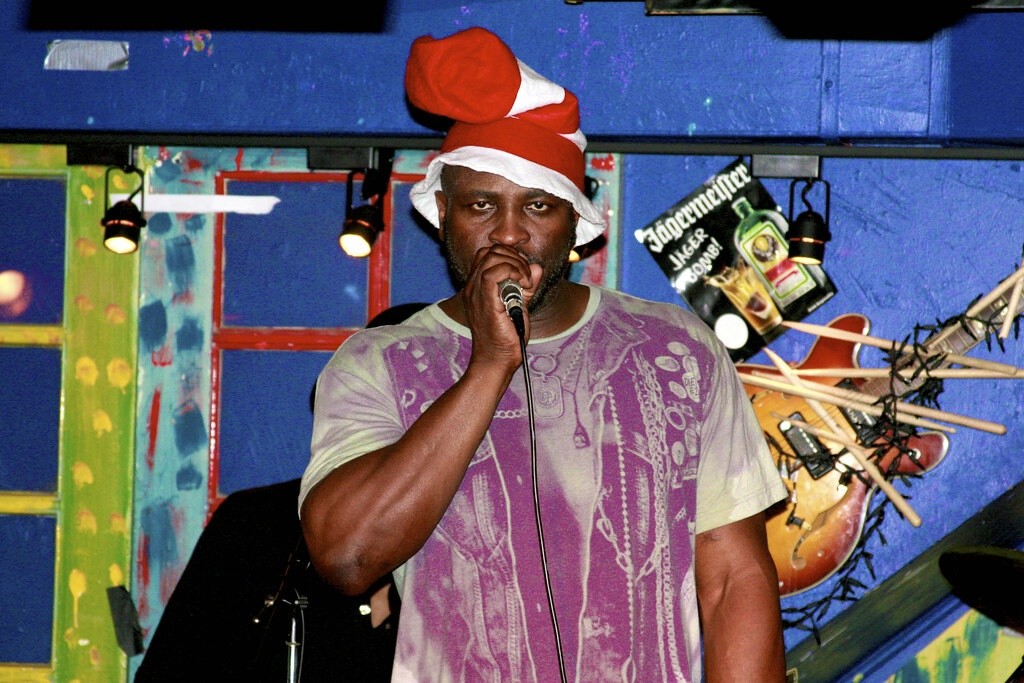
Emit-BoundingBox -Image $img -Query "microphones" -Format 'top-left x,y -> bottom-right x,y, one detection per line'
498,278 -> 525,335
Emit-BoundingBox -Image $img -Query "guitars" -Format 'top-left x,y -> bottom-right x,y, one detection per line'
729,252 -> 1024,598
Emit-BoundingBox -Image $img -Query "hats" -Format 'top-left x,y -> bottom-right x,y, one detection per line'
404,27 -> 608,248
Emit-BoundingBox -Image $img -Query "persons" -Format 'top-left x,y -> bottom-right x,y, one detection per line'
133,302 -> 432,683
297,26 -> 791,683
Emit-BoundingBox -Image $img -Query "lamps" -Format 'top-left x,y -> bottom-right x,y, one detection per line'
788,211 -> 825,264
339,205 -> 382,257
103,202 -> 141,255
